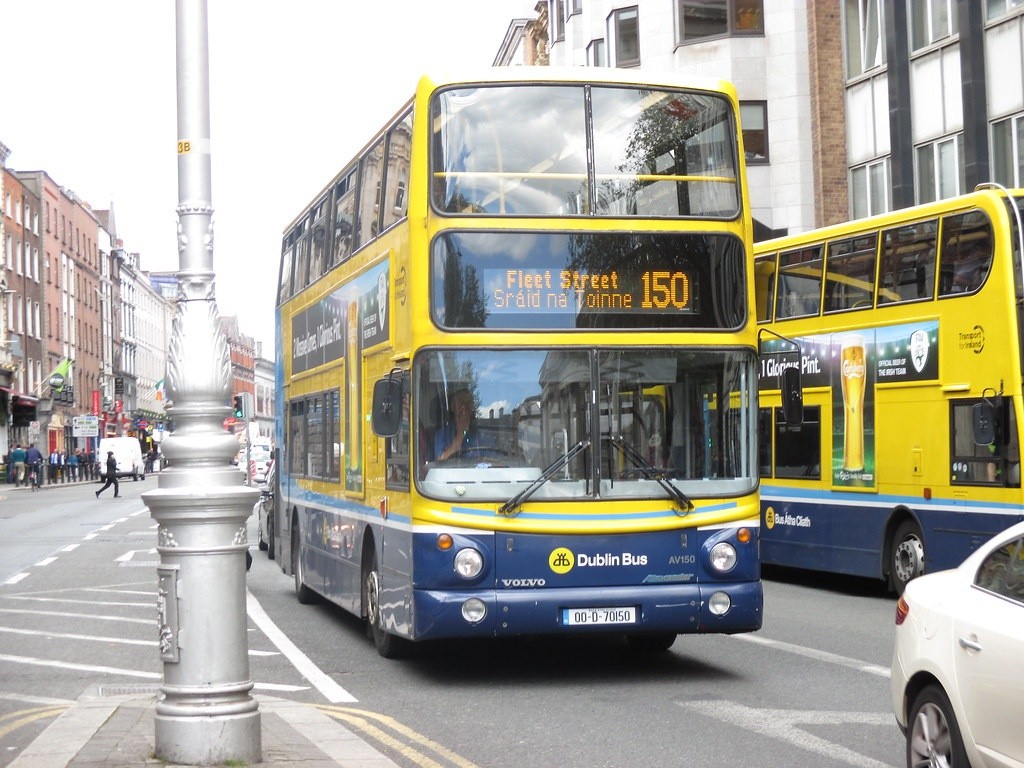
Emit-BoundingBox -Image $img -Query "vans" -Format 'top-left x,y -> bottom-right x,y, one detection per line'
99,436 -> 146,483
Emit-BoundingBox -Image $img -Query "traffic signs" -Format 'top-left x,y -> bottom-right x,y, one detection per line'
73,416 -> 98,437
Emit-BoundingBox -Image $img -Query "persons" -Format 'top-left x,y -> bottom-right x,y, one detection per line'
955,239 -> 991,292
144,448 -> 157,474
987,438 -> 1000,481
787,279 -> 819,316
7,443 -> 101,485
95,451 -> 122,498
435,387 -> 498,461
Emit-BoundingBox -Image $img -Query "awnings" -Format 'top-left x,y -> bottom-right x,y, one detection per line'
0,387 -> 39,406
145,428 -> 171,442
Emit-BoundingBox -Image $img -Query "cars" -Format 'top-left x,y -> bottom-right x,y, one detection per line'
890,522 -> 1024,768
236,434 -> 275,560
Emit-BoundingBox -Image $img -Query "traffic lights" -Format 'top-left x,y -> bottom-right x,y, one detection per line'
233,393 -> 244,419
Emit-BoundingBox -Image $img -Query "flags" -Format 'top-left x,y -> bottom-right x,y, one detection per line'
154,378 -> 166,400
49,357 -> 73,392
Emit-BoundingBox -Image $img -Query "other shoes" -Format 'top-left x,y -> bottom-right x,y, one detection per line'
96,492 -> 99,498
114,495 -> 121,498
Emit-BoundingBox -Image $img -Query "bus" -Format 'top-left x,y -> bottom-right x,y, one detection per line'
276,67 -> 803,662
615,183 -> 1024,596
